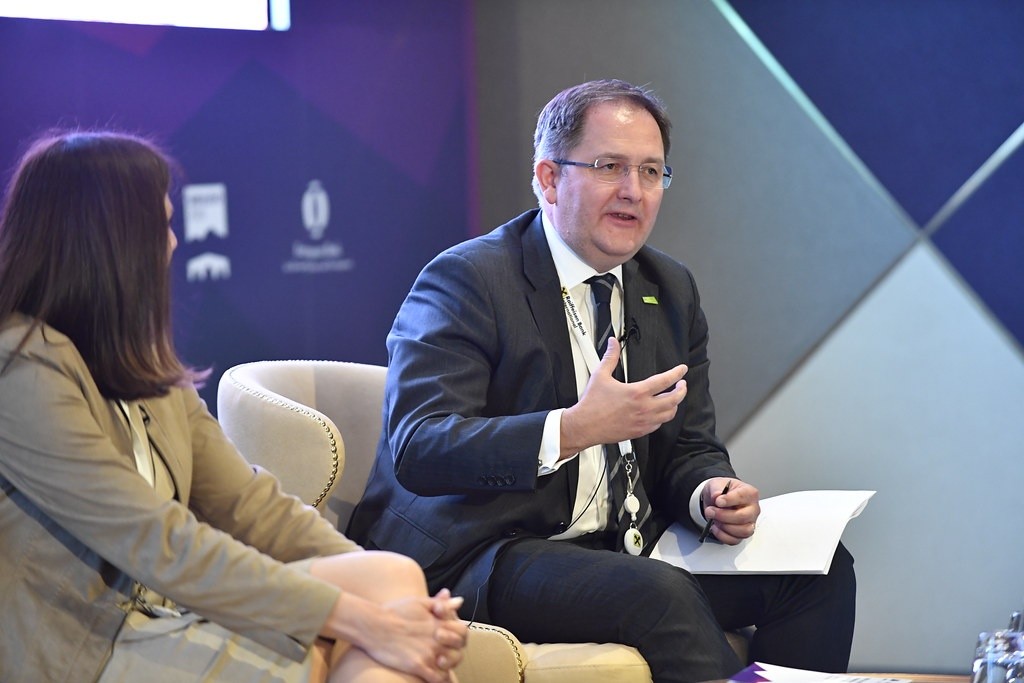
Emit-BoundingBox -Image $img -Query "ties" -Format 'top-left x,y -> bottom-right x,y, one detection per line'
582,273 -> 658,556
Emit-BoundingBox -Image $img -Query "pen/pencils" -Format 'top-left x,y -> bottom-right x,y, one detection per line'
699,481 -> 732,544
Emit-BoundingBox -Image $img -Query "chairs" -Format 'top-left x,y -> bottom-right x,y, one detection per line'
217,357 -> 749,683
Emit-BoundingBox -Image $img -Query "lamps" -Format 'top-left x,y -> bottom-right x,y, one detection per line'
0,0 -> 270,32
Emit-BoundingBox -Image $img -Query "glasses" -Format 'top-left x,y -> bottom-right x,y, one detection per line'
548,157 -> 673,190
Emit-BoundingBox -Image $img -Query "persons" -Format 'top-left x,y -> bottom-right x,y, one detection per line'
344,79 -> 856,683
0,134 -> 468,683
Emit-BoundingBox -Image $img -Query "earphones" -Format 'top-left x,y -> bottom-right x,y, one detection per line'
138,403 -> 150,425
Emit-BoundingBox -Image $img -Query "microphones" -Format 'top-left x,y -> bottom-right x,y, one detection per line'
618,315 -> 642,343
